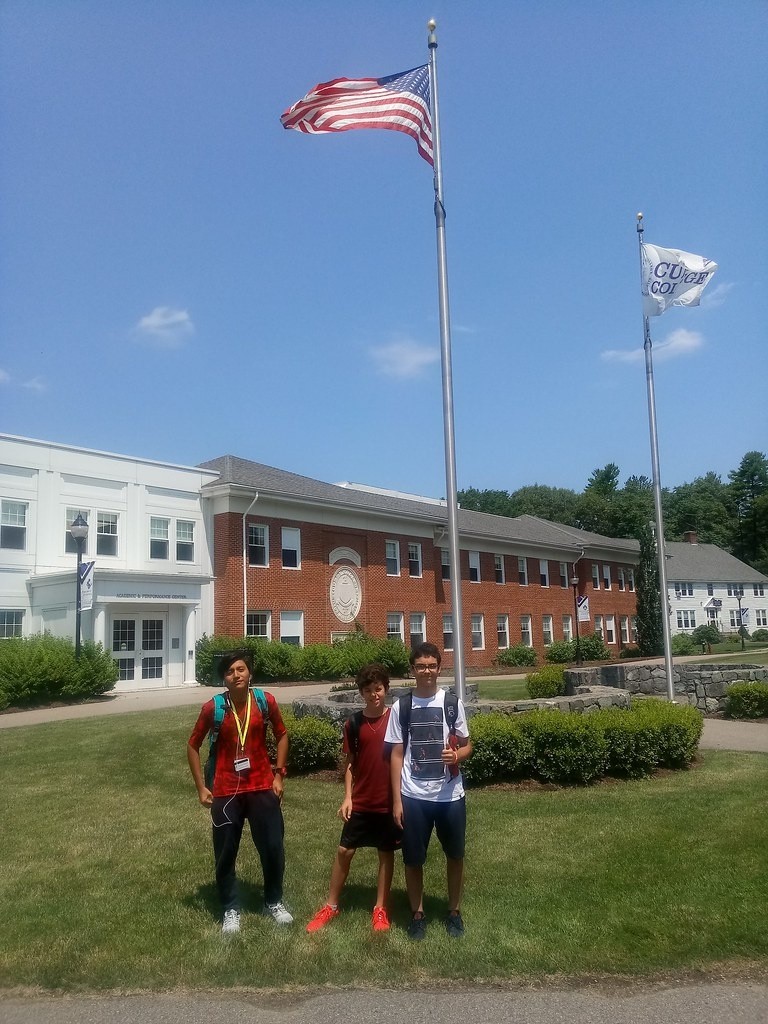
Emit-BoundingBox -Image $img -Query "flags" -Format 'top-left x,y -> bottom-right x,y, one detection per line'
279,62 -> 434,167
641,242 -> 717,316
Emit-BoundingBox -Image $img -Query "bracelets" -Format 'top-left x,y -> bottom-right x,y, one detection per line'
454,751 -> 457,764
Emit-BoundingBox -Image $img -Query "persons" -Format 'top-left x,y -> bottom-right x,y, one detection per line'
187,652 -> 293,932
306,664 -> 401,933
383,643 -> 473,943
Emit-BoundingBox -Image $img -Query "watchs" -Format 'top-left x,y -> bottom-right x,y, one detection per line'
275,767 -> 287,776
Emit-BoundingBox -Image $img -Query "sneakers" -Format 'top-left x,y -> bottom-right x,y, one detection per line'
266,900 -> 293,924
306,904 -> 339,932
221,909 -> 240,934
372,907 -> 390,931
408,911 -> 426,940
446,910 -> 464,937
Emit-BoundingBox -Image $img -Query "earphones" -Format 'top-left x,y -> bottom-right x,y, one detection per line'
248,676 -> 251,683
223,680 -> 225,686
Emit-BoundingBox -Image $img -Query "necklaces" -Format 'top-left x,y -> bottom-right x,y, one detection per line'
364,709 -> 386,733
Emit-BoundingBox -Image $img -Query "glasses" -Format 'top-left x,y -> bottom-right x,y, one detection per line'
412,662 -> 441,674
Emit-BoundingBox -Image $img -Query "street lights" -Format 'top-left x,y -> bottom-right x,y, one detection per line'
736,590 -> 747,651
570,572 -> 584,667
70,510 -> 90,657
